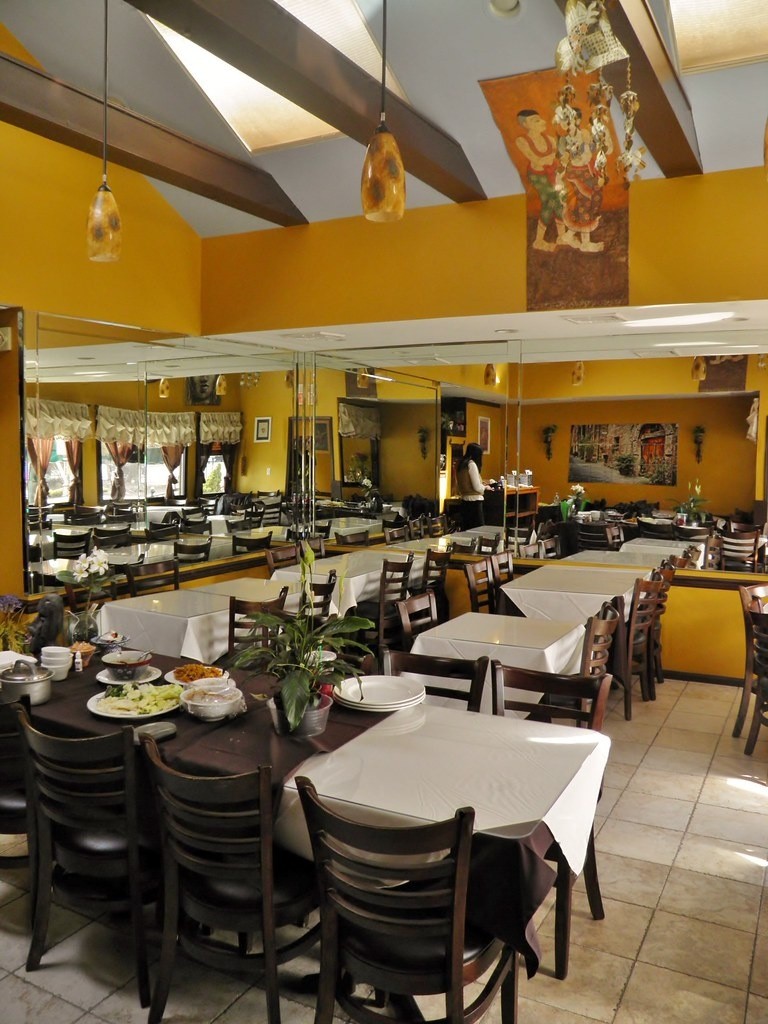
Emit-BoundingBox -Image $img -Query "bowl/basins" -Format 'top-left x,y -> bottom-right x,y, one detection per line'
608,514 -> 624,520
101,651 -> 153,681
41,646 -> 72,680
179,678 -> 243,721
68,646 -> 96,667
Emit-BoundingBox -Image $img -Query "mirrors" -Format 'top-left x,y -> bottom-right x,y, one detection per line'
506,352 -> 768,530
338,402 -> 380,488
22,310 -> 438,439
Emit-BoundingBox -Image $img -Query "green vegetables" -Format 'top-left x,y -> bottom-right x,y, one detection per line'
105,682 -> 183,714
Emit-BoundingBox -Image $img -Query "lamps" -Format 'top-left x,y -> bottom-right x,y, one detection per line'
85,0 -> 121,263
484,356 -> 707,387
361,0 -> 406,221
158,368 -> 373,398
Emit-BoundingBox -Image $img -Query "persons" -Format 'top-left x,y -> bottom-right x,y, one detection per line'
455,444 -> 492,528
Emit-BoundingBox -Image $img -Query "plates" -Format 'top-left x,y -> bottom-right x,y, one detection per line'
333,675 -> 426,712
96,666 -> 162,684
605,519 -> 626,522
87,691 -> 180,718
332,706 -> 426,736
622,520 -> 637,524
164,667 -> 229,685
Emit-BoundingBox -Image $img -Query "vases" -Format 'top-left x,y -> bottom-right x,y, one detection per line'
266,692 -> 333,739
2,630 -> 25,654
62,610 -> 101,656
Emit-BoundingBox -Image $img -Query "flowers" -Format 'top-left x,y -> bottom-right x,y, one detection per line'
0,594 -> 30,626
568,481 -> 588,499
665,474 -> 709,523
55,546 -> 127,597
217,537 -> 373,729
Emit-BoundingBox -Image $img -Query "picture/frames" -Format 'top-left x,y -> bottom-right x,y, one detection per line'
310,420 -> 331,454
478,416 -> 491,455
254,416 -> 271,443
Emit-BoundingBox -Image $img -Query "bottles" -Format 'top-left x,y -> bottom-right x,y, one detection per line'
554,491 -> 559,505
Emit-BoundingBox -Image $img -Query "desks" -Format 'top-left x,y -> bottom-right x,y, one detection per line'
95,590 -> 284,667
618,538 -> 705,570
403,612 -> 585,725
0,634 -> 280,933
386,538 -> 492,588
187,577 -> 311,648
176,687 -> 613,980
27,497 -> 408,576
499,563 -> 656,690
506,485 -> 540,540
270,551 -> 441,653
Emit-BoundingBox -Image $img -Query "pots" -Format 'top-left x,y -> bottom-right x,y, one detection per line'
0,659 -> 55,706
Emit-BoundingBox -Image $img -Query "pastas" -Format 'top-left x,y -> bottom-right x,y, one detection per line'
174,663 -> 222,683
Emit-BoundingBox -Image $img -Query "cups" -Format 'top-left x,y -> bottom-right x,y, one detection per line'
304,650 -> 337,698
590,511 -> 600,521
677,513 -> 687,527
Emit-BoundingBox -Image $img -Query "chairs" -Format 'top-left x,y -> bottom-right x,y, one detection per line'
0,498 -> 768,1024
